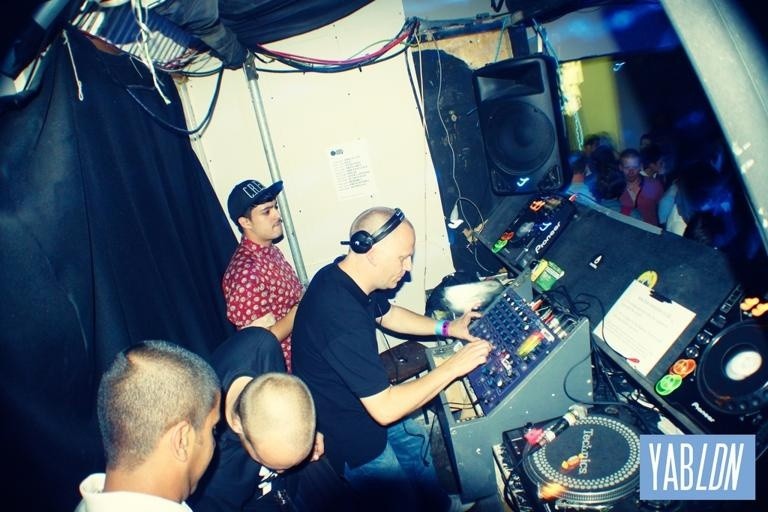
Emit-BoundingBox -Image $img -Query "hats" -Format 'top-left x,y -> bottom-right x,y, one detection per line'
227,179 -> 283,221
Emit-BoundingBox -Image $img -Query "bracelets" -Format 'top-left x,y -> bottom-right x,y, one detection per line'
435,318 -> 443,337
443,319 -> 449,338
293,301 -> 301,307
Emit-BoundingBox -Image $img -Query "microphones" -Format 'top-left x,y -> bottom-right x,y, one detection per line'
530,402 -> 588,456
341,241 -> 351,245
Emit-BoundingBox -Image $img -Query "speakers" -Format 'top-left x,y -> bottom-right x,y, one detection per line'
473,55 -> 566,195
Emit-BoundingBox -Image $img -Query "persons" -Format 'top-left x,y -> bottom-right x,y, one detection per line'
290,206 -> 496,510
184,326 -> 327,511
72,338 -> 221,512
221,179 -> 306,374
567,130 -> 720,248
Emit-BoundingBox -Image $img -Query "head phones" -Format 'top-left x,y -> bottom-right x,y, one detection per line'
351,209 -> 405,253
